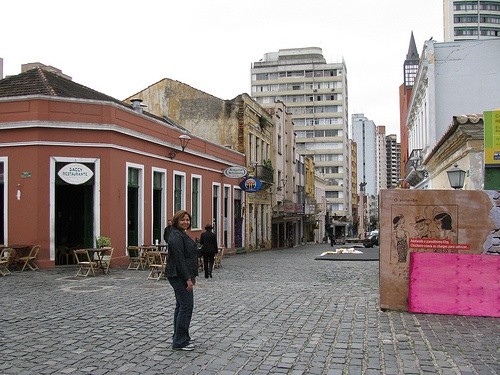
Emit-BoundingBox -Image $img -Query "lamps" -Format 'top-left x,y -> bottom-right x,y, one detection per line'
247,161 -> 258,172
446,163 -> 466,190
411,156 -> 424,169
169,134 -> 191,158
278,178 -> 287,191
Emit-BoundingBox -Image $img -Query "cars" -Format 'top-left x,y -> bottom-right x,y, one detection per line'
363,230 -> 379,248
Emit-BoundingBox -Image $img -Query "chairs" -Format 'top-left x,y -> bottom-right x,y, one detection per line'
19,245 -> 40,271
56,246 -> 114,277
0,248 -> 12,277
127,246 -> 225,281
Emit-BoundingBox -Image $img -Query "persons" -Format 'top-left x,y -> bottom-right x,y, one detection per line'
329,233 -> 335,247
163,220 -> 172,244
165,211 -> 199,351
200,224 -> 219,278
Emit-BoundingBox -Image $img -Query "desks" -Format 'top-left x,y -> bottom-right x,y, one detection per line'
143,245 -> 168,250
0,244 -> 32,269
79,248 -> 110,274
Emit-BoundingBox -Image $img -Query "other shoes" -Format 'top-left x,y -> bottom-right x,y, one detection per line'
172,343 -> 195,351
205,274 -> 212,278
189,338 -> 194,343
